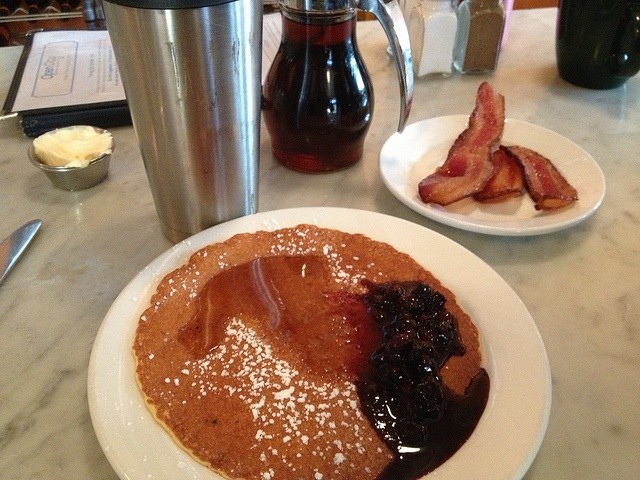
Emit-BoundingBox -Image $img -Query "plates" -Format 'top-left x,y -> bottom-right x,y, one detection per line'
86,207 -> 552,480
378,115 -> 607,236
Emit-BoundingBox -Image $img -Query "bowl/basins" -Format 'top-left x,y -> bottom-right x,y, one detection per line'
26,124 -> 114,192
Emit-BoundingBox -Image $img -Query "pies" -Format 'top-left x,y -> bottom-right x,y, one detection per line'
127,222 -> 490,480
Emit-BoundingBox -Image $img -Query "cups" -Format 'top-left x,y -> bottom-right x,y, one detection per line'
555,0 -> 640,89
101,0 -> 264,244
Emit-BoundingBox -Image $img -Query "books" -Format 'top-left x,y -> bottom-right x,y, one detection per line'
3,28 -> 133,138
260,13 -> 283,94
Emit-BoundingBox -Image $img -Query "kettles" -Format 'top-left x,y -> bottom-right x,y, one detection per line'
261,0 -> 414,174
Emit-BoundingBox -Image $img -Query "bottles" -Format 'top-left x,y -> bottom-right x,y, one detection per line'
408,1 -> 459,81
452,0 -> 505,76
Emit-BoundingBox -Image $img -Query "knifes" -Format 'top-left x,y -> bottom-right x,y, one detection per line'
0,219 -> 43,286
